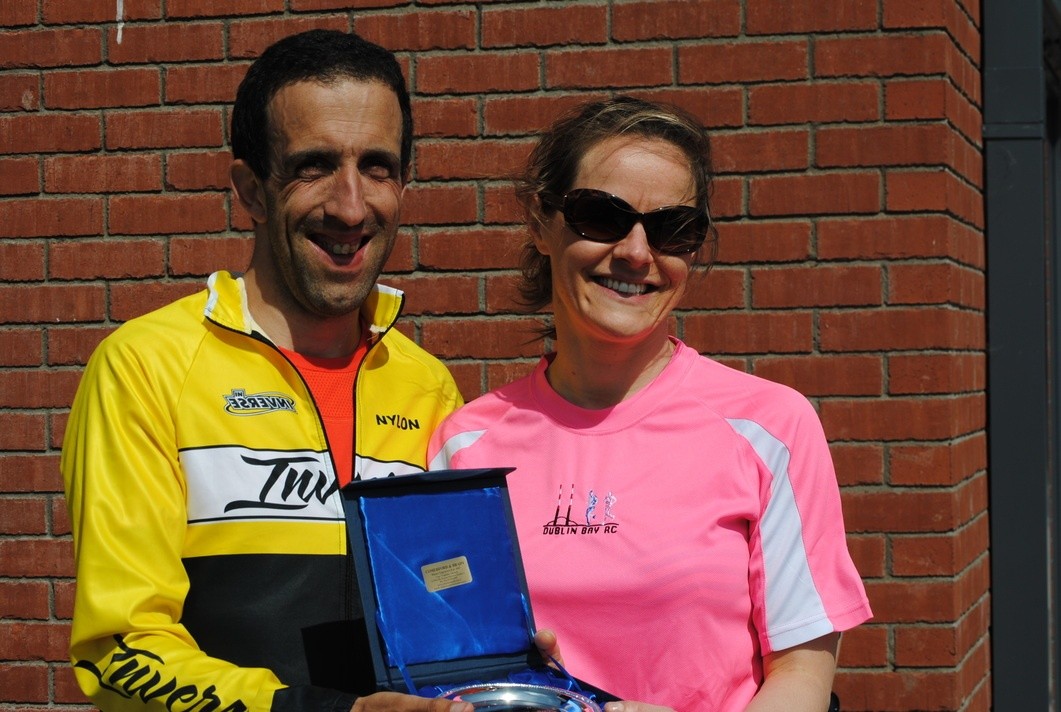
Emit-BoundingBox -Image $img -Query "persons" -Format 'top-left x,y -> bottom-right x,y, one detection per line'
59,28 -> 566,712
426,94 -> 875,712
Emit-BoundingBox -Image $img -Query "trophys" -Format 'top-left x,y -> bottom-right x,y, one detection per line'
434,682 -> 601,712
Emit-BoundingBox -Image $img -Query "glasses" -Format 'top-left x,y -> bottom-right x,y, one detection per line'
538,188 -> 708,255
